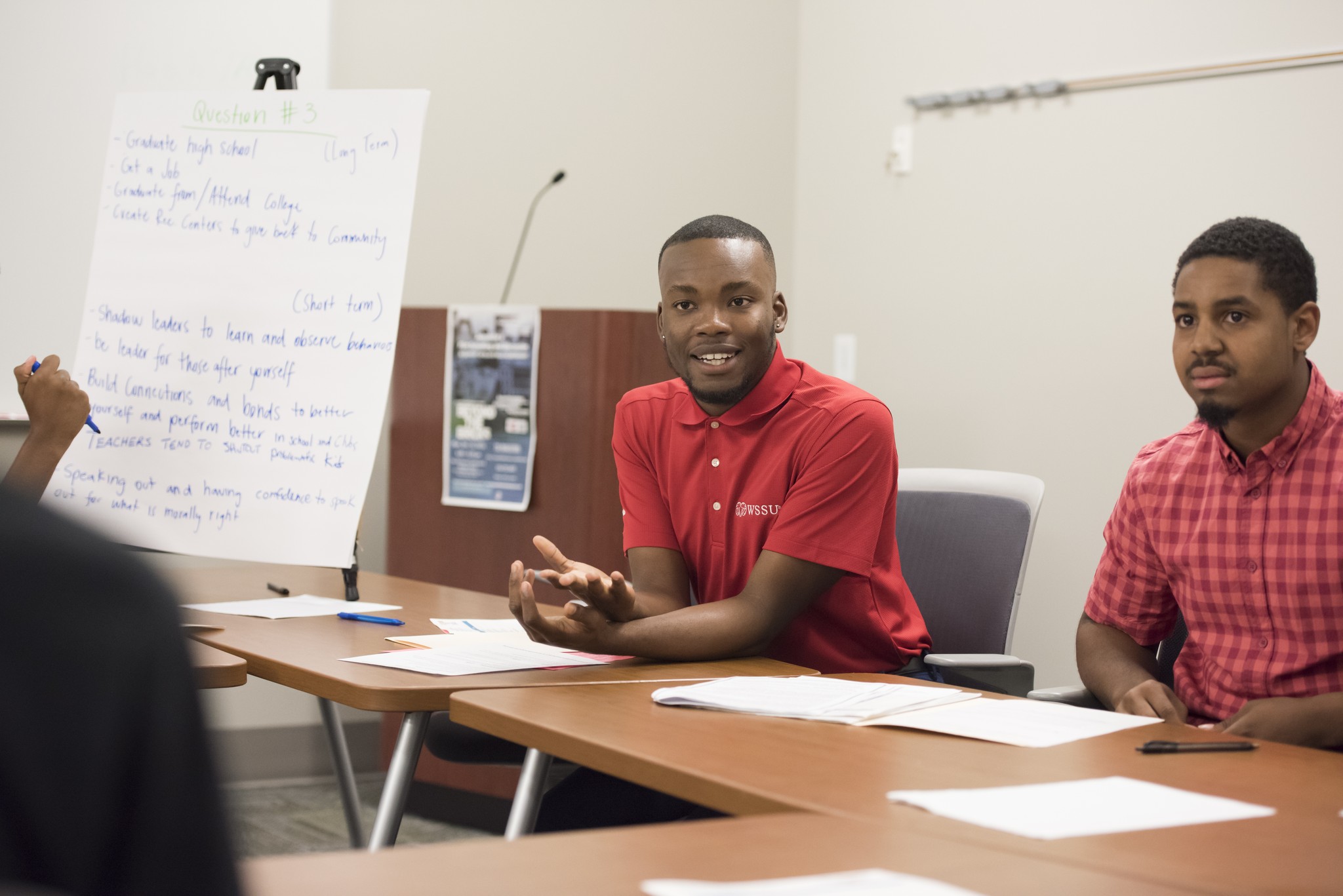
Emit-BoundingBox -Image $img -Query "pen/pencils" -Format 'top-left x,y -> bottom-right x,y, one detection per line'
30,360 -> 101,434
267,583 -> 290,595
1135,741 -> 1260,753
337,612 -> 406,626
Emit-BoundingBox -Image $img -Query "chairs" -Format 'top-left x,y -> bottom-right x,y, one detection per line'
892,466 -> 1046,701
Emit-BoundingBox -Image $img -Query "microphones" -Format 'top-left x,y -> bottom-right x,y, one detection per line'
501,168 -> 564,304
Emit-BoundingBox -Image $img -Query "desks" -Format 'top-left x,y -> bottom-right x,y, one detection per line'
125,550 -> 1343,896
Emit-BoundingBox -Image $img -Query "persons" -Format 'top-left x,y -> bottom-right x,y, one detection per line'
508,215 -> 943,836
1075,217 -> 1343,754
0,354 -> 249,896
